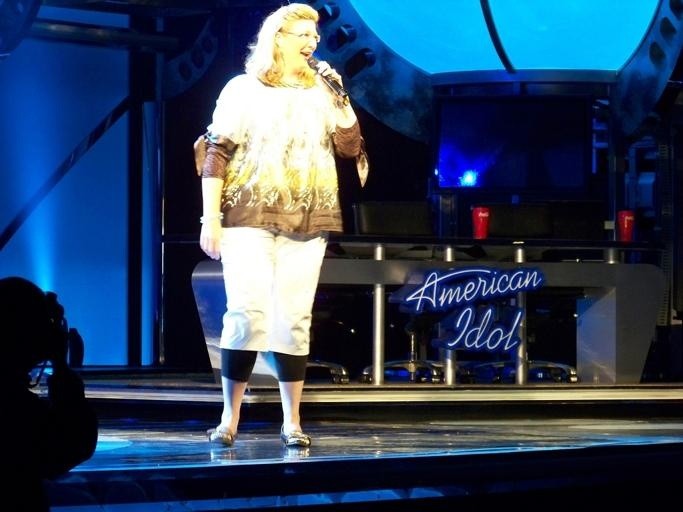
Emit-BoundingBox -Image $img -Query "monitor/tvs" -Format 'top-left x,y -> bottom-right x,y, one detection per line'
428,84 -> 611,206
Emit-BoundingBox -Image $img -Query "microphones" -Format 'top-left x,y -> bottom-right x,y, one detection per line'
305,56 -> 347,100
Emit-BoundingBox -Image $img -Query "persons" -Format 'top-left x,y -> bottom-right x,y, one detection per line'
200,2 -> 361,448
0,276 -> 99,511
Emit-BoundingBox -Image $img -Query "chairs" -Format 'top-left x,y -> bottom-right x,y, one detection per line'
473,207 -> 578,382
303,306 -> 350,385
354,199 -> 459,382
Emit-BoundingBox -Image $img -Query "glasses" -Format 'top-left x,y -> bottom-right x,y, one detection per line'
286,32 -> 320,44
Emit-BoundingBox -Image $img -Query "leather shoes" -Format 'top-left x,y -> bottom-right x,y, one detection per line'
208,427 -> 239,445
279,423 -> 310,446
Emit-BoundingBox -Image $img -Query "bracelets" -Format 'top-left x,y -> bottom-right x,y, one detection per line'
200,213 -> 224,225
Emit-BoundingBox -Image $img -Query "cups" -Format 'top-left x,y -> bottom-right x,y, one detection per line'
617,209 -> 634,243
471,207 -> 490,240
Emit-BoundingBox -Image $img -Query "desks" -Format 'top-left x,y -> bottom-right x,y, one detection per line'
191,239 -> 668,386
